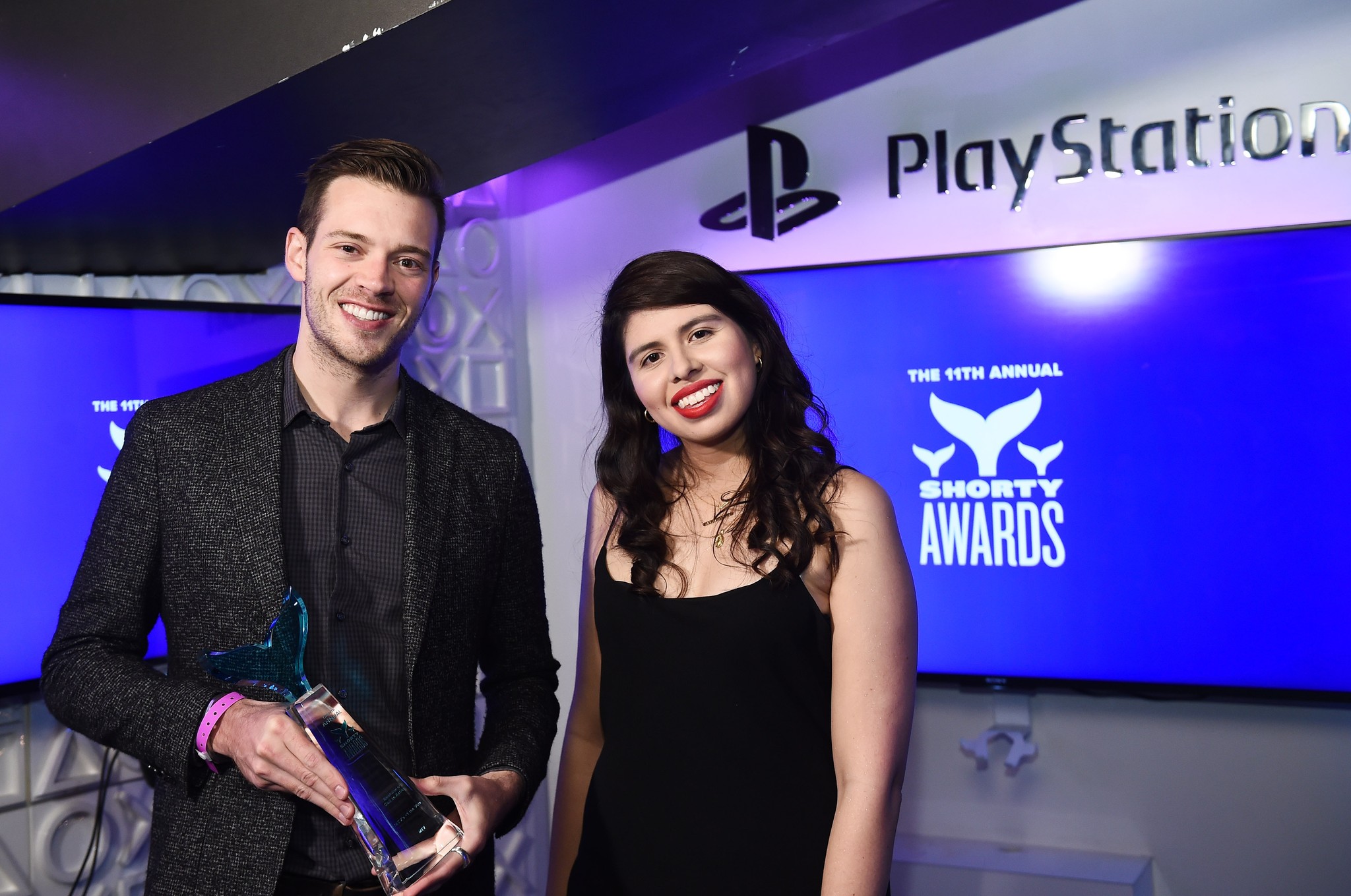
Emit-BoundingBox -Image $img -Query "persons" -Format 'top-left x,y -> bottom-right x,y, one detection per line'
40,141 -> 563,896
545,249 -> 916,896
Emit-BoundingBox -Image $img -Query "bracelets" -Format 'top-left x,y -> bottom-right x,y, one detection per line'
195,692 -> 247,774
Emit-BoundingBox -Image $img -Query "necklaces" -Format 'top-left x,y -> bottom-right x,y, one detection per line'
679,465 -> 737,548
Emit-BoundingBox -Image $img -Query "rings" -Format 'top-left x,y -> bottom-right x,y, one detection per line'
449,846 -> 470,868
